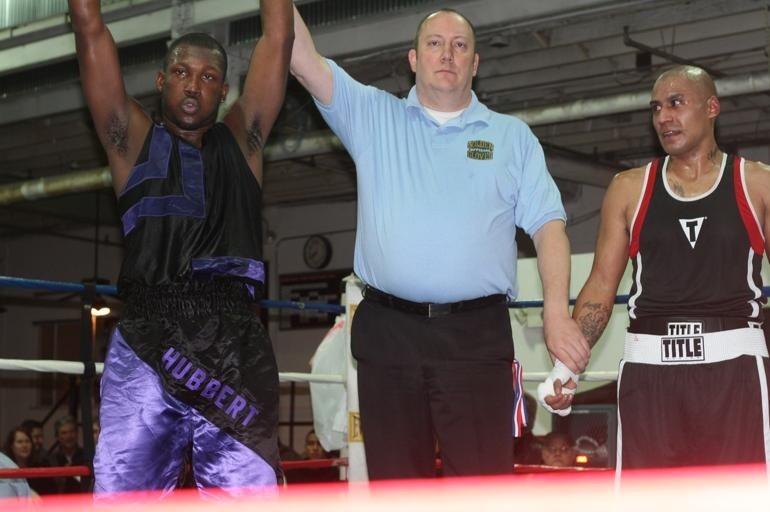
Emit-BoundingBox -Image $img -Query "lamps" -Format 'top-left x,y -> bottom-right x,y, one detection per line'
90,190 -> 111,322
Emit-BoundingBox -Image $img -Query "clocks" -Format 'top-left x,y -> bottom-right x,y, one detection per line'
301,233 -> 332,270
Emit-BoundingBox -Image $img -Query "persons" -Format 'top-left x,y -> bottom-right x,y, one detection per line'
66,0 -> 296,510
538,64 -> 769,488
8,428 -> 39,493
277,435 -> 303,461
92,416 -> 101,446
288,428 -> 339,484
45,415 -> 92,491
20,420 -> 61,493
286,0 -> 588,503
541,431 -> 574,466
515,390 -> 544,468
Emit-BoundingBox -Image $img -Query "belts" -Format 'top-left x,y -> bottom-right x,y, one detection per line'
364,284 -> 508,319
